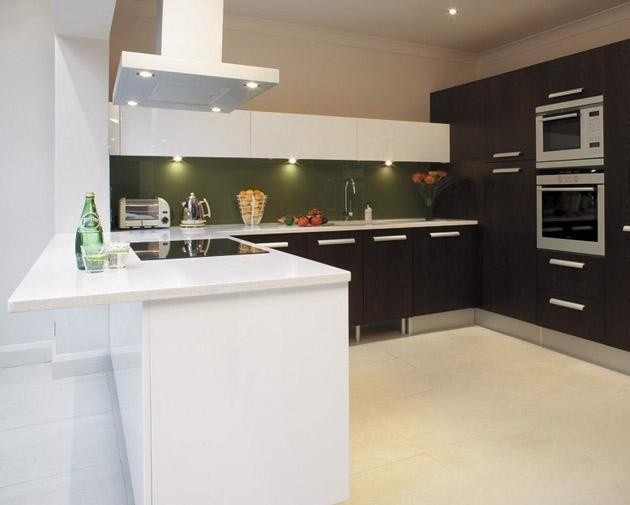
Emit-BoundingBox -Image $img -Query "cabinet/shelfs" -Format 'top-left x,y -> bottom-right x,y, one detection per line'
538,248 -> 604,369
306,229 -> 411,344
109,101 -> 451,163
426,77 -> 483,228
484,63 -> 539,343
232,225 -> 306,255
619,36 -> 630,377
540,40 -> 618,103
413,225 -> 478,315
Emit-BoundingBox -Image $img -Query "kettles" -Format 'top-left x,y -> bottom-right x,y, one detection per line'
180,230 -> 212,258
178,191 -> 211,228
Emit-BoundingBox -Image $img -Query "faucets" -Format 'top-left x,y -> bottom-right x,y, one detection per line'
344,177 -> 357,220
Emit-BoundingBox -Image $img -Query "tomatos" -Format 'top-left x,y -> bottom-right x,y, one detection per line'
311,217 -> 323,226
298,217 -> 308,227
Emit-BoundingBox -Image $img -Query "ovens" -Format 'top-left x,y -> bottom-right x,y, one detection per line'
535,95 -> 606,257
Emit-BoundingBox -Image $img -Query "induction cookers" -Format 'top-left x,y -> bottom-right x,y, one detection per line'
131,237 -> 270,262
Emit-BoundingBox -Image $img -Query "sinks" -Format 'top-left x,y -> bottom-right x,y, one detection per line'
322,222 -> 376,226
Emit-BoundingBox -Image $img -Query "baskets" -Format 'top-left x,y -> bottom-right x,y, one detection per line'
235,194 -> 268,226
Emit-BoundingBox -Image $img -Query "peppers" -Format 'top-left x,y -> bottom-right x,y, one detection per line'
284,213 -> 294,225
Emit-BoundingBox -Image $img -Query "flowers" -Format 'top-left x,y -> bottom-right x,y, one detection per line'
411,170 -> 453,199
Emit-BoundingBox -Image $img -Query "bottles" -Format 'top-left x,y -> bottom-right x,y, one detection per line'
74,191 -> 104,270
363,203 -> 373,225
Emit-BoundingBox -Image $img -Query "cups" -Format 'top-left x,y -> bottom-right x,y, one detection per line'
80,245 -> 107,274
105,239 -> 131,268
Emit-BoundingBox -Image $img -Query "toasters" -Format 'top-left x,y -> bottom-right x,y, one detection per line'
120,197 -> 172,230
128,229 -> 170,259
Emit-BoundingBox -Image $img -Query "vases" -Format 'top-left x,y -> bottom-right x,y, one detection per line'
424,193 -> 437,220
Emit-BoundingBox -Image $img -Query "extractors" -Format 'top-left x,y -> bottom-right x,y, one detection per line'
112,1 -> 280,114
